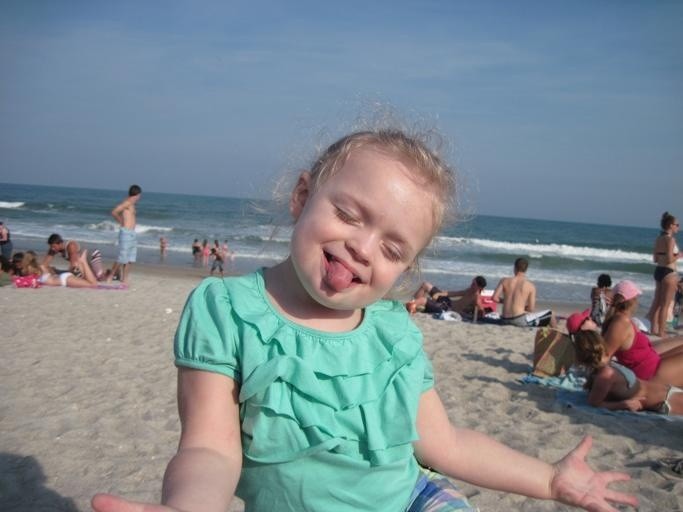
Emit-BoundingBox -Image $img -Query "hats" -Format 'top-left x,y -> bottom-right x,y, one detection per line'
567,308 -> 592,333
613,280 -> 642,301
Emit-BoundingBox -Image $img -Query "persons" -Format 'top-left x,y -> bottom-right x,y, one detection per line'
88,114 -> 643,512
649,211 -> 681,339
412,276 -> 487,320
568,273 -> 681,416
492,257 -> 556,328
0,184 -> 230,290
